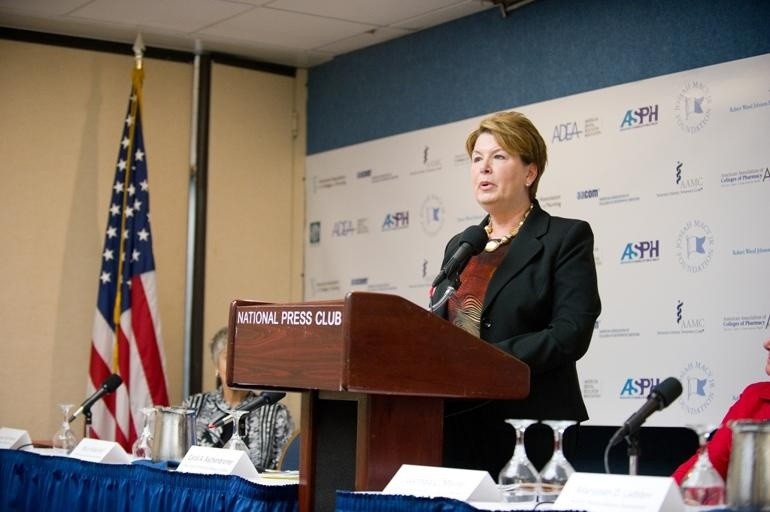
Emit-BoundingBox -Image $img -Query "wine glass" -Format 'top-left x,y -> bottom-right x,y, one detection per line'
129,406 -> 153,458
538,419 -> 580,505
499,417 -> 540,507
51,402 -> 77,454
682,422 -> 727,507
221,406 -> 251,459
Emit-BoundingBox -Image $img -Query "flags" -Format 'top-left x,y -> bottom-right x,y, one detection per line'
83,70 -> 169,454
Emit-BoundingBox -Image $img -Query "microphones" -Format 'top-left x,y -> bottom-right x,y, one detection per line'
611,377 -> 683,445
215,391 -> 287,426
432,226 -> 489,287
68,373 -> 123,423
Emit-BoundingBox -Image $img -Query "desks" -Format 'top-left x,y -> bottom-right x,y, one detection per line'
1,440 -> 299,512
336,488 -> 588,512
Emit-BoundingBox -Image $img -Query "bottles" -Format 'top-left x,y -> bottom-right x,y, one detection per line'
727,417 -> 769,512
152,405 -> 199,467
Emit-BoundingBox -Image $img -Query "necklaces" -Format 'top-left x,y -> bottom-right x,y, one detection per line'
482,200 -> 539,253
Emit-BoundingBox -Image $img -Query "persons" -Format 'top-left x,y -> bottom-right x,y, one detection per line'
177,324 -> 295,471
428,112 -> 605,484
669,338 -> 770,501
